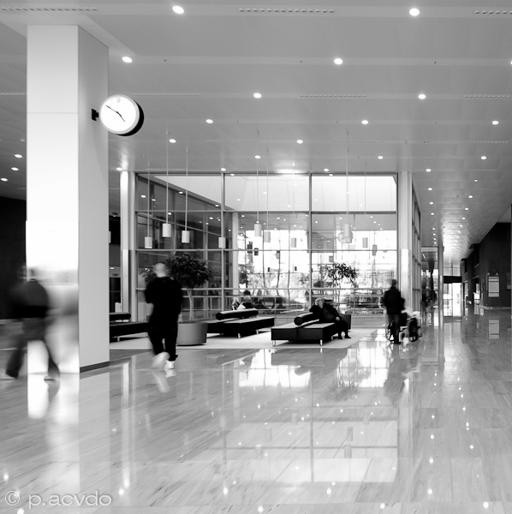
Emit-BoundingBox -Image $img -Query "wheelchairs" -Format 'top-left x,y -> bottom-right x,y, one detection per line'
384,311 -> 423,342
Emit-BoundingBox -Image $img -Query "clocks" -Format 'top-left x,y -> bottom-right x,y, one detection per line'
90,94 -> 142,136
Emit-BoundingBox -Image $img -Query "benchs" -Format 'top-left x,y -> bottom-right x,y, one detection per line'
200,308 -> 351,347
109,313 -> 149,344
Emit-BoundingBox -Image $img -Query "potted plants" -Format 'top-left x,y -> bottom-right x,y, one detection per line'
163,253 -> 211,347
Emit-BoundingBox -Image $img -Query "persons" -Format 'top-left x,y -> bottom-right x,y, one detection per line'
401,298 -> 422,339
422,289 -> 437,309
0,266 -> 61,383
382,279 -> 405,344
231,289 -> 285,312
309,297 -> 351,339
143,260 -> 184,371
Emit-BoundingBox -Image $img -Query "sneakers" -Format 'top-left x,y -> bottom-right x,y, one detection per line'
338,334 -> 351,339
0,368 -> 15,380
152,352 -> 176,369
